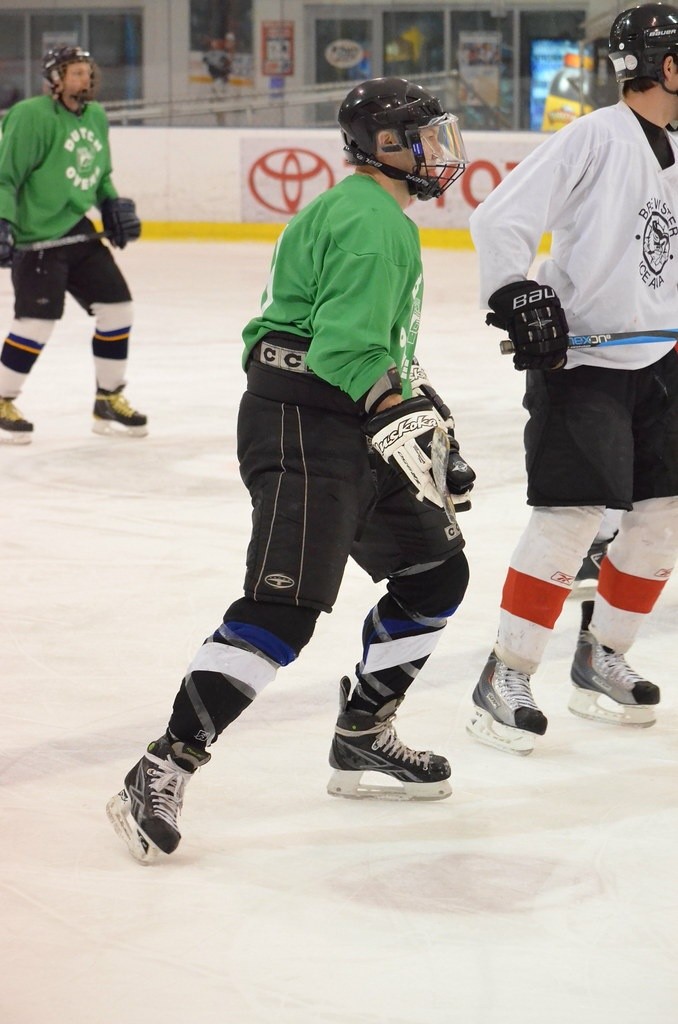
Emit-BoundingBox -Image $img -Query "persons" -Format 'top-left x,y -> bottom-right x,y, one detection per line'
0,45 -> 147,445
470,43 -> 499,65
202,39 -> 231,103
464,4 -> 678,756
105,76 -> 478,866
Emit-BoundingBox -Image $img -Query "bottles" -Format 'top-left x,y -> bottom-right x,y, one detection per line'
541,52 -> 595,134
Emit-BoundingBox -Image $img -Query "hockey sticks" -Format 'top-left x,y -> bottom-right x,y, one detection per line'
499,329 -> 678,356
13,230 -> 114,253
429,425 -> 457,528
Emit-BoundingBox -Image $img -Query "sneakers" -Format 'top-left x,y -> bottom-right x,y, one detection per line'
566,602 -> 660,725
324,674 -> 455,802
106,731 -> 214,864
468,648 -> 549,751
89,386 -> 147,437
0,395 -> 34,446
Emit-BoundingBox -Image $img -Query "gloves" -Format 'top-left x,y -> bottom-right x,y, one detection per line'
101,197 -> 141,248
485,282 -> 569,372
362,396 -> 472,514
0,219 -> 14,268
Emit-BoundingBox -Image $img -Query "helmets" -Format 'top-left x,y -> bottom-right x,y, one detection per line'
42,45 -> 101,105
608,2 -> 678,83
338,77 -> 465,199
